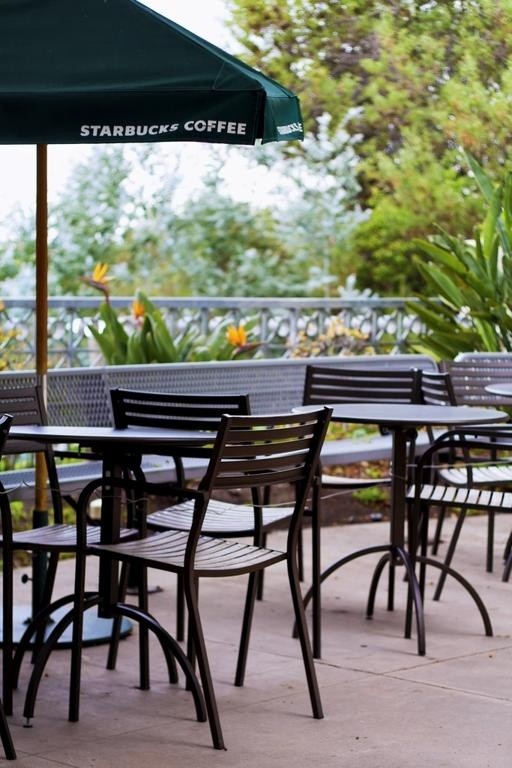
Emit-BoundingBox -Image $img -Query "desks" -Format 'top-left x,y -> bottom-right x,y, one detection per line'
5,425 -> 219,731
290,404 -> 509,657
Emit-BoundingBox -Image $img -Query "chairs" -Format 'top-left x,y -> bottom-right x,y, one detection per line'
1,386 -> 148,617
110,388 -> 309,601
250,362 -> 423,576
406,428 -> 512,601
66,406 -> 334,751
408,365 -> 512,555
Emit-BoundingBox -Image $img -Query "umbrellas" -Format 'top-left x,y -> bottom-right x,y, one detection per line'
0,0 -> 305,528
